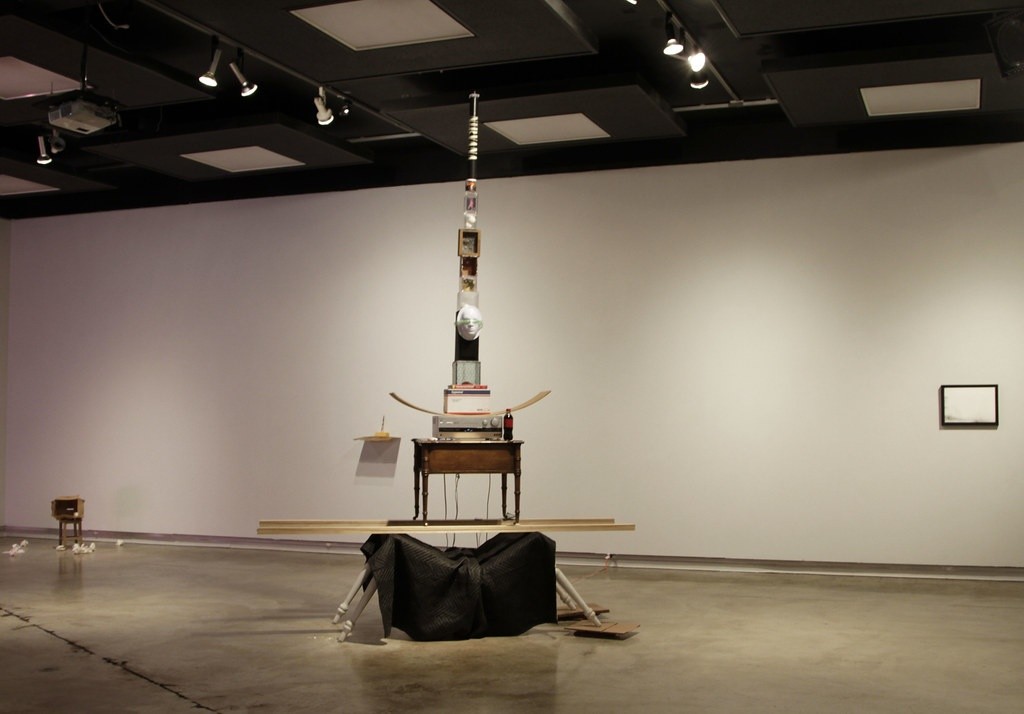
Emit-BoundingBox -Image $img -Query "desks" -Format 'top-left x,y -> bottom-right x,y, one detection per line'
411,439 -> 525,523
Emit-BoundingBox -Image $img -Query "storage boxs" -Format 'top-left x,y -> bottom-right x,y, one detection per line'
51,495 -> 86,519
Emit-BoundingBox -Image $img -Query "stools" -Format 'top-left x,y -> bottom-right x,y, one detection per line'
58,519 -> 83,548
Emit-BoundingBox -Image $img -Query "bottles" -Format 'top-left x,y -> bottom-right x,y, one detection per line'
504,409 -> 513,440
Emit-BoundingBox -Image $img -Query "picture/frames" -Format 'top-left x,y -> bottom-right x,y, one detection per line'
940,384 -> 999,427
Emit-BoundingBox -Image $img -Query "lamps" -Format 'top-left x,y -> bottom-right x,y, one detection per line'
34,121 -> 52,165
199,43 -> 224,86
662,12 -> 683,55
229,50 -> 257,97
313,86 -> 334,125
